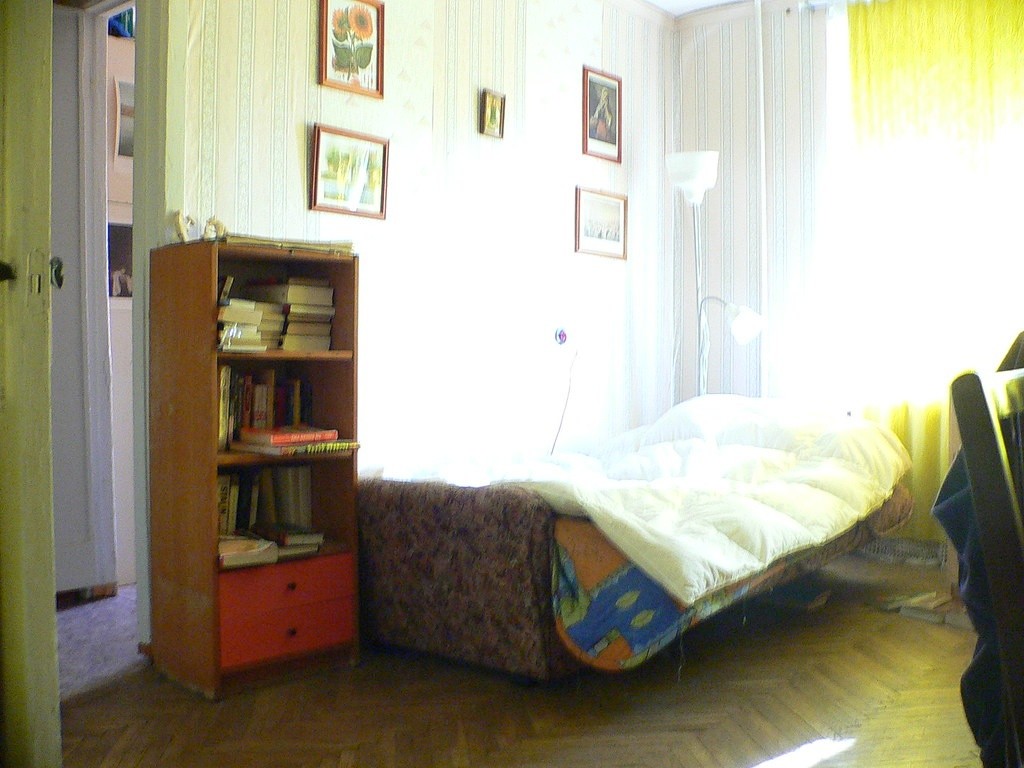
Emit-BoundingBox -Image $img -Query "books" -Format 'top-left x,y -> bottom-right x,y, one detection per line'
218,365 -> 360,455
220,274 -> 335,352
217,463 -> 326,568
770,579 -> 976,631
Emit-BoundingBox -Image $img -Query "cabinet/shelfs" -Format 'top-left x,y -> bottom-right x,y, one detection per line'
145,239 -> 361,703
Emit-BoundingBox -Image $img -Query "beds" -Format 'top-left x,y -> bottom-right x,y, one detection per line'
357,396 -> 915,685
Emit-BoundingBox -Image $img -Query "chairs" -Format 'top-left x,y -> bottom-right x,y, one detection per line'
950,367 -> 1024,768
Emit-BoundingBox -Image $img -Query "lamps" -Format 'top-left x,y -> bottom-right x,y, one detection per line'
665,150 -> 740,396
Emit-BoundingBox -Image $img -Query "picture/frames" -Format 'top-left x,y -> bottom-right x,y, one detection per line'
581,64 -> 623,164
318,0 -> 385,100
308,121 -> 391,220
574,184 -> 628,261
480,87 -> 506,139
